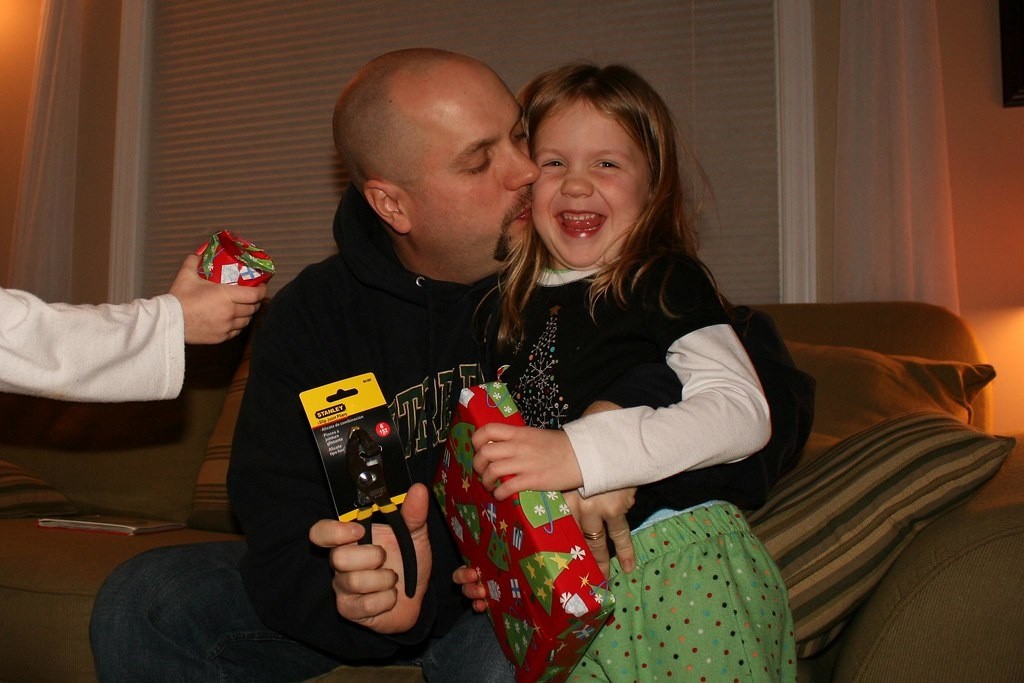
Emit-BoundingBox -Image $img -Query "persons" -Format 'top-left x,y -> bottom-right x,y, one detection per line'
451,61 -> 799,683
88,47 -> 818,683
0,253 -> 268,404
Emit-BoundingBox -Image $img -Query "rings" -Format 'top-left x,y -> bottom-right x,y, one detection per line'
583,528 -> 605,541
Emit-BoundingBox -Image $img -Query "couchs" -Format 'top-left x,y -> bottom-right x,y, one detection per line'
0,301 -> 1024,683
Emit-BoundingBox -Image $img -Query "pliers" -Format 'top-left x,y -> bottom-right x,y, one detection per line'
344,429 -> 418,598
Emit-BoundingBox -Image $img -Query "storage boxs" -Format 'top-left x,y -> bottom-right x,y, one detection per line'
433,381 -> 616,683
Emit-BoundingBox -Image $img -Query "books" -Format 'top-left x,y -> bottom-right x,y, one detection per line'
37,514 -> 186,537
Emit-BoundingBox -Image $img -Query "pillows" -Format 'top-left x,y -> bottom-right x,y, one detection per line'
0,459 -> 75,519
192,333 -> 255,533
773,340 -> 996,487
742,411 -> 1015,659
